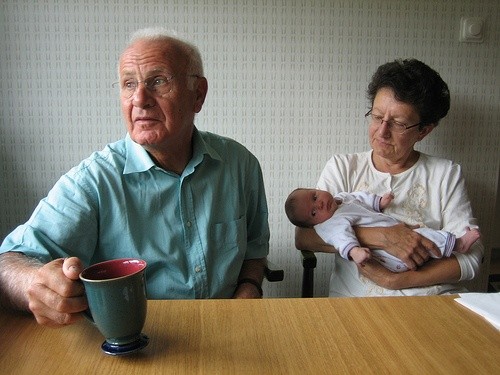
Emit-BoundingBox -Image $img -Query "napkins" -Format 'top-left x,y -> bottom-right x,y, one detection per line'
453,293 -> 500,330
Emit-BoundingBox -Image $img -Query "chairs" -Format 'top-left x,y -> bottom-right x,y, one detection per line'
266,260 -> 284,282
300,250 -> 317,298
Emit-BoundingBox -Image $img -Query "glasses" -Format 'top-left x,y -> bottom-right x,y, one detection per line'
112,71 -> 200,99
365,110 -> 422,134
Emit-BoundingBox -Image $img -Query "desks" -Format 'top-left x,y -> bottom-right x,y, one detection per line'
0,293 -> 500,375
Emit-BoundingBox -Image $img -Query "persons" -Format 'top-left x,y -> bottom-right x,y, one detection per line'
0,29 -> 270,320
294,59 -> 480,297
284,188 -> 481,268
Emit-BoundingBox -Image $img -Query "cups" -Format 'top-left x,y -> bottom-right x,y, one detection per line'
79,257 -> 149,355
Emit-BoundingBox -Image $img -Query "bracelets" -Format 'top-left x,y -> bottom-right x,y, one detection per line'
238,277 -> 265,296
